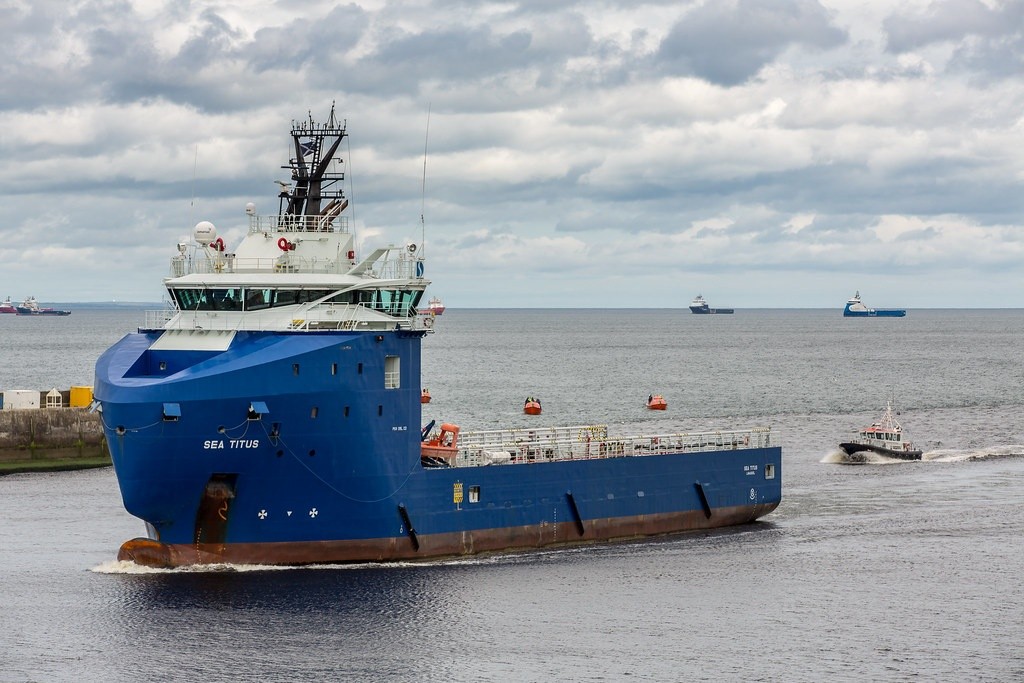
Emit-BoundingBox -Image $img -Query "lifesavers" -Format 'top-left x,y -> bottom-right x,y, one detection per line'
278,238 -> 288,249
424,317 -> 433,327
216,238 -> 224,251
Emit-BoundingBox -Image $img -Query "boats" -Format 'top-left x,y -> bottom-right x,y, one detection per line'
523,397 -> 542,415
839,401 -> 923,460
16,295 -> 71,316
689,294 -> 734,314
645,394 -> 667,411
842,290 -> 906,317
0,295 -> 17,313
417,297 -> 446,315
421,388 -> 432,403
90,99 -> 782,570
